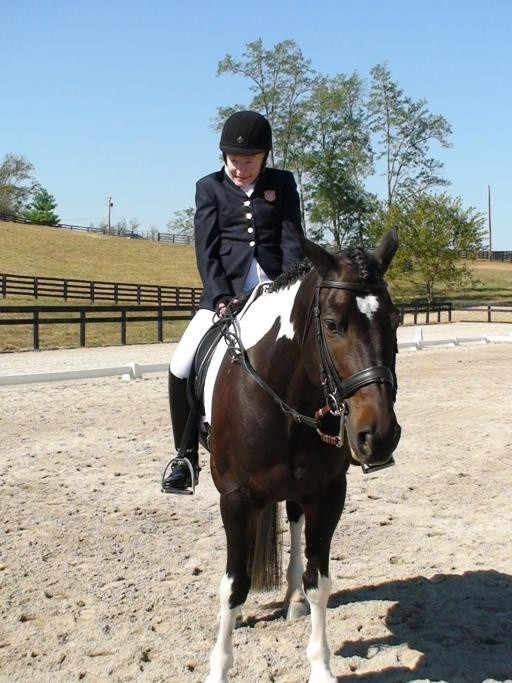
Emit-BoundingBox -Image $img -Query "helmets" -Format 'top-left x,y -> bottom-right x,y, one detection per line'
219,111 -> 272,156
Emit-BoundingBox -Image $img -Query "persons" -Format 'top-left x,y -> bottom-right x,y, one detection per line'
161,111 -> 306,490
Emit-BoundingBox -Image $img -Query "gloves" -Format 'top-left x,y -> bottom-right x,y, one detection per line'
215,295 -> 238,318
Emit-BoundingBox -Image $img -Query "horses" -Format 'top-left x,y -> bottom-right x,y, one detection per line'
177,225 -> 402,683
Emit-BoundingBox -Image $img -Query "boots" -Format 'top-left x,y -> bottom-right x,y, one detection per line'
162,364 -> 201,489
